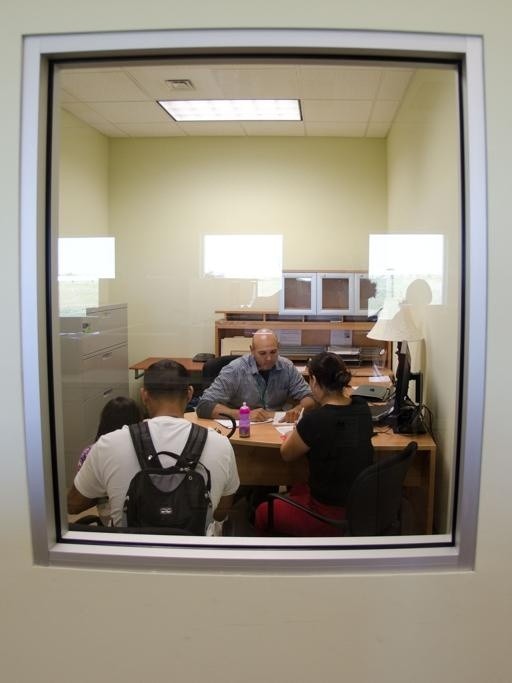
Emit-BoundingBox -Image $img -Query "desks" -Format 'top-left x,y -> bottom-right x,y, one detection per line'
183,409 -> 439,537
350,373 -> 393,390
129,355 -> 206,386
214,308 -> 394,370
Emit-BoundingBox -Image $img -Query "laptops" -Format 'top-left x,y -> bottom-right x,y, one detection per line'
350,384 -> 388,402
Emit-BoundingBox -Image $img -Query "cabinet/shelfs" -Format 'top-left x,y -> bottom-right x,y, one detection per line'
60,301 -> 130,493
279,269 -> 395,318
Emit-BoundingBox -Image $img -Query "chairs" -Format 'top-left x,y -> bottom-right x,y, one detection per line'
68,513 -> 202,534
263,440 -> 420,537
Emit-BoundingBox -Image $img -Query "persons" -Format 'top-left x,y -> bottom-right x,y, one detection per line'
67,358 -> 241,537
195,328 -> 320,422
254,350 -> 374,536
76,392 -> 143,527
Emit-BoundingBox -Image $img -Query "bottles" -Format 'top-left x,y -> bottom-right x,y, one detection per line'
239,401 -> 251,438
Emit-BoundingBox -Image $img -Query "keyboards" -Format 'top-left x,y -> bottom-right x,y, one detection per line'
368,405 -> 394,422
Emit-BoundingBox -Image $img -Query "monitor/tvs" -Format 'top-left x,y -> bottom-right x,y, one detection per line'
393,341 -> 427,433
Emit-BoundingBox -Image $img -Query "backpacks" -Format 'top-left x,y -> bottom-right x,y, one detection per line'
124,422 -> 213,536
